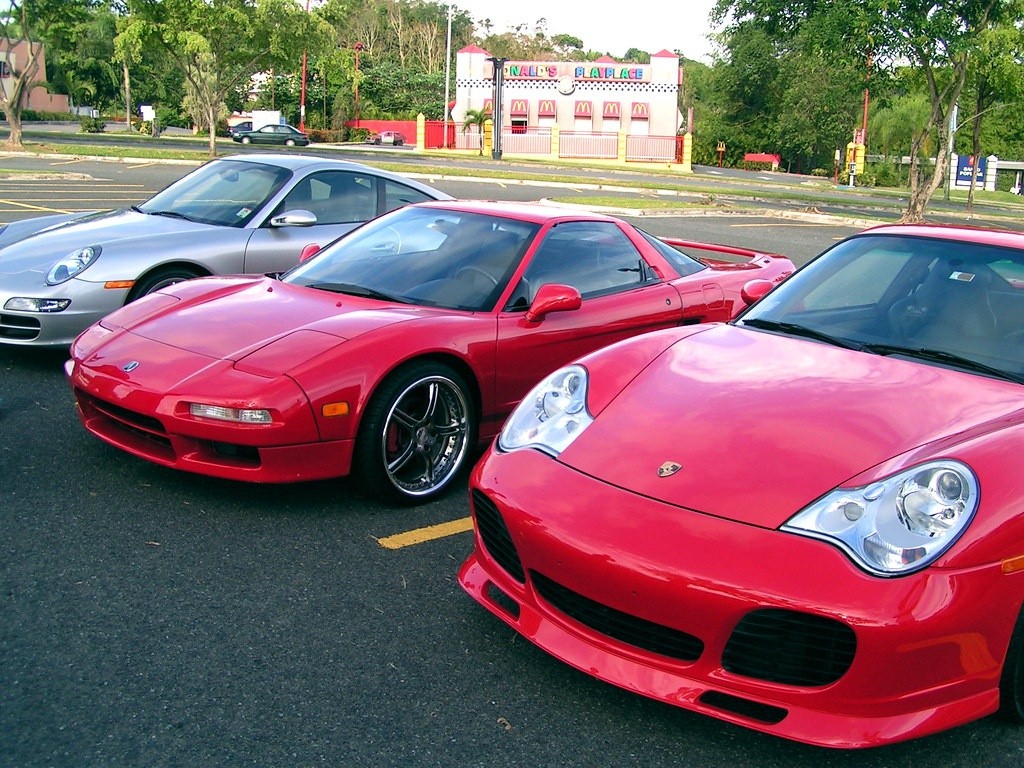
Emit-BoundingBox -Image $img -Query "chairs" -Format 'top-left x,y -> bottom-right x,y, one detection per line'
265,175 -> 311,228
529,240 -> 605,302
876,260 -> 1011,360
458,231 -> 522,295
328,175 -> 356,224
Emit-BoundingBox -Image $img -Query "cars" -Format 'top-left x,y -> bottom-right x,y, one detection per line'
226,122 -> 252,137
232,124 -> 310,147
366,130 -> 407,146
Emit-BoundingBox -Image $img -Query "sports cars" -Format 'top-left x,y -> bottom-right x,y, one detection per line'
453,211 -> 1024,751
64,201 -> 805,503
1,152 -> 515,380
